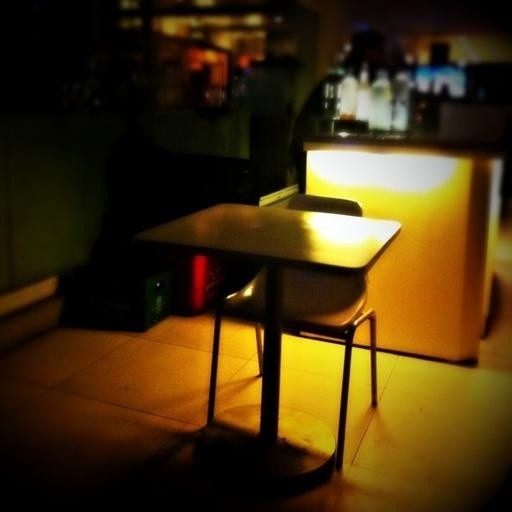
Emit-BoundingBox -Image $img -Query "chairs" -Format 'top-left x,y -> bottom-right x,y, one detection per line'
207,192 -> 378,470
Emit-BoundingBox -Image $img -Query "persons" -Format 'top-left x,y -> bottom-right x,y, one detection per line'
338,28 -> 387,88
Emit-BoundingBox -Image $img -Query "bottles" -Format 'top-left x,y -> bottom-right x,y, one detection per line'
339,63 -> 412,132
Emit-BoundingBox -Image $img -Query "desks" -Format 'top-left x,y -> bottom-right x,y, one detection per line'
132,202 -> 401,497
